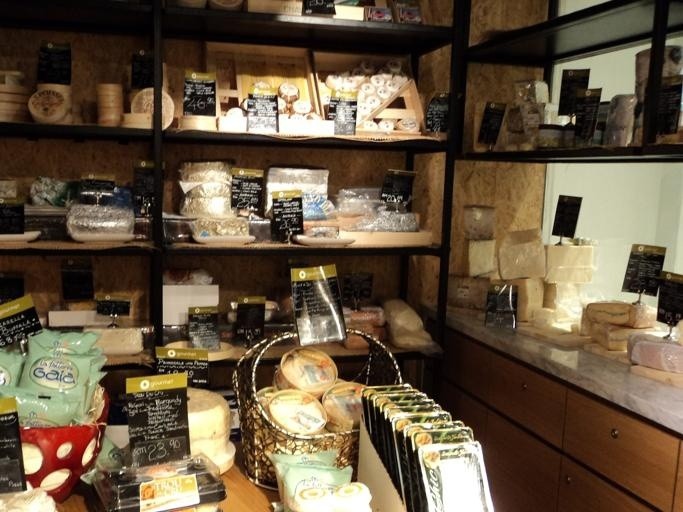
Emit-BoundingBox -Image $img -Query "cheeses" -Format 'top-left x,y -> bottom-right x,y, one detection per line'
448,228 -> 683,388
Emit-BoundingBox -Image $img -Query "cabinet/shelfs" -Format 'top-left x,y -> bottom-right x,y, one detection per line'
557,382 -> 682,511
450,0 -> 682,164
446,325 -> 567,512
2,1 -> 454,387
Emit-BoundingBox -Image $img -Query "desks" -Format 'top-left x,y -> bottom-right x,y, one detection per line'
0,433 -> 279,510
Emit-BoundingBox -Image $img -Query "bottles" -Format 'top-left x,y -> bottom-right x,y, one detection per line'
95,82 -> 123,127
462,204 -> 496,240
37,82 -> 74,123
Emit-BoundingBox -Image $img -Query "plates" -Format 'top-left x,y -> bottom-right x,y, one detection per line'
192,234 -> 254,245
0,229 -> 41,244
70,231 -> 135,246
293,233 -> 354,246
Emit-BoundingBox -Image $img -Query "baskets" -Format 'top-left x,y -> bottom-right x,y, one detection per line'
231,327 -> 403,492
